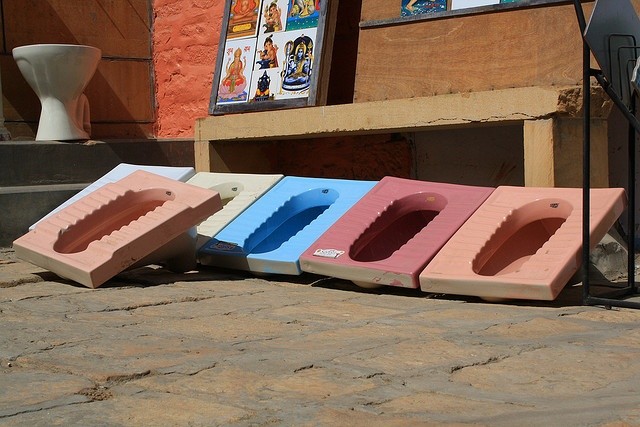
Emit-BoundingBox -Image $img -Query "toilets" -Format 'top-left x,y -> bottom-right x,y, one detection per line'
11,44 -> 103,142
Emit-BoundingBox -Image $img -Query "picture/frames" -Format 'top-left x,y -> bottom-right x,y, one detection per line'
208,0 -> 339,116
